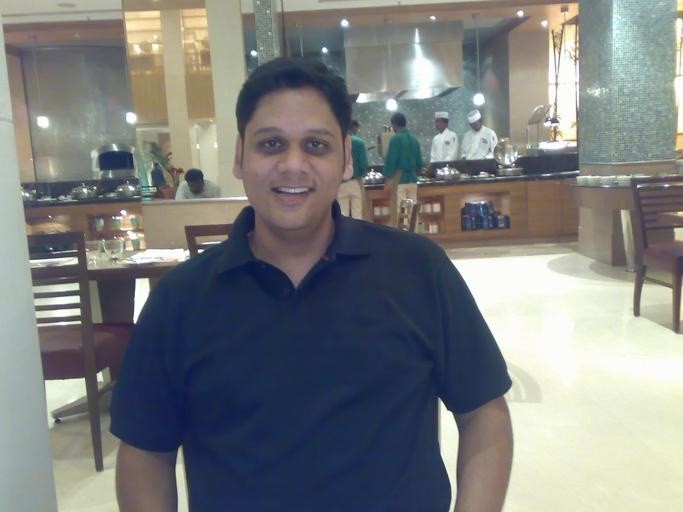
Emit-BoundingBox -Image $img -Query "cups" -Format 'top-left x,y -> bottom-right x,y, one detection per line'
81,240 -> 101,265
102,239 -> 125,262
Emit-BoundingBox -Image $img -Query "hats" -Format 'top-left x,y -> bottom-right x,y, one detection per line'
467,110 -> 481,123
435,112 -> 448,118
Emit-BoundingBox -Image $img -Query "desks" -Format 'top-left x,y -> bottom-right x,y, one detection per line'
27,247 -> 204,423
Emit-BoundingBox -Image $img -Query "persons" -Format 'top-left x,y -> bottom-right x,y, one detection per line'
112,58 -> 514,508
460,109 -> 499,161
429,110 -> 459,163
380,112 -> 426,234
150,161 -> 167,190
174,169 -> 220,200
337,121 -> 367,221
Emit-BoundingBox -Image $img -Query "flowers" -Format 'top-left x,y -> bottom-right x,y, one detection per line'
165,166 -> 184,174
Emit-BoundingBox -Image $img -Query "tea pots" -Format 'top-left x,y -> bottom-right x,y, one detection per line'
71,182 -> 98,199
362,169 -> 384,185
21,192 -> 34,202
434,164 -> 460,180
115,180 -> 135,197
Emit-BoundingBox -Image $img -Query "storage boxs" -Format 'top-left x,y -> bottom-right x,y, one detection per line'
460,200 -> 509,230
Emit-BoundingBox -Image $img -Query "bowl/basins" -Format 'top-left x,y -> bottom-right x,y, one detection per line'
573,174 -> 650,187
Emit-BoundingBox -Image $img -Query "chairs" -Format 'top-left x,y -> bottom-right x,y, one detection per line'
396,197 -> 417,233
628,175 -> 682,332
184,223 -> 233,259
85,210 -> 141,251
26,232 -> 132,470
